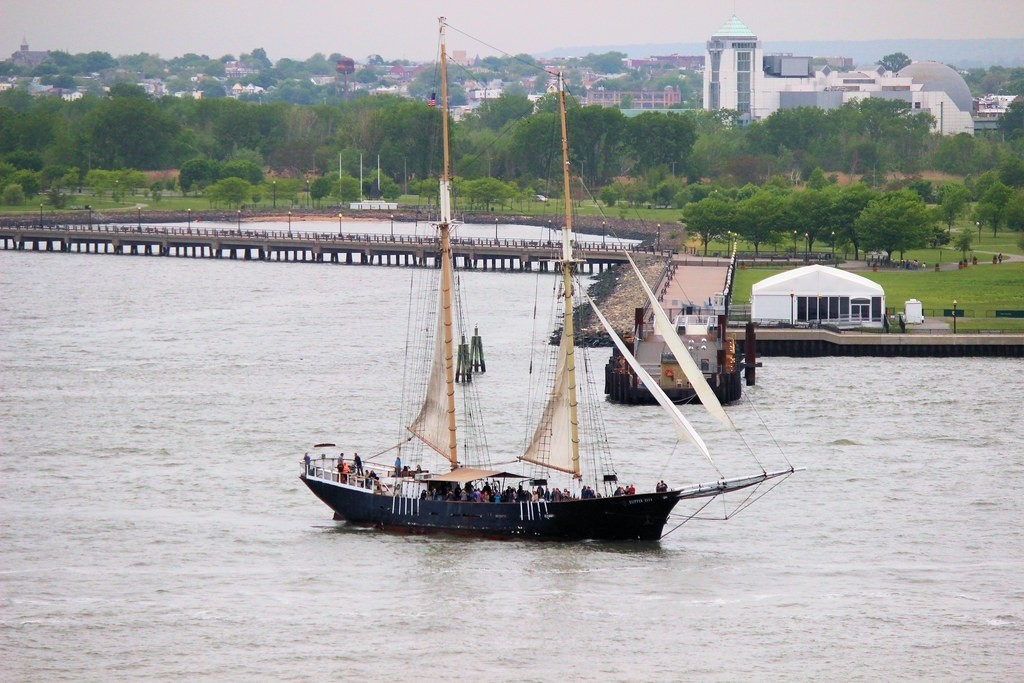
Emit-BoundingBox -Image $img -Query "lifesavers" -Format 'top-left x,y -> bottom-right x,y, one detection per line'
665,369 -> 674,377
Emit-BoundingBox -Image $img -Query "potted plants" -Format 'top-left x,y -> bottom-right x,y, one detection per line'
935,263 -> 940,271
963,259 -> 968,267
958,261 -> 964,269
739,261 -> 745,270
992,255 -> 997,264
973,255 -> 978,264
873,263 -> 878,271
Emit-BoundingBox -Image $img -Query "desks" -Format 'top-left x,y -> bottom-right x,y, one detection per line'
354,477 -> 381,490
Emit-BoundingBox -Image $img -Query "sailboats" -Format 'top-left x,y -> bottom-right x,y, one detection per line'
297,15 -> 808,544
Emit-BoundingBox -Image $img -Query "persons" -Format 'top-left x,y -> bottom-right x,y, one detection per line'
582,485 -> 601,498
656,482 -> 667,493
338,453 -> 343,464
998,253 -> 1003,263
354,453 -> 364,476
417,465 -> 421,471
483,482 -> 491,494
465,481 -> 473,492
395,457 -> 401,471
614,485 -> 635,496
304,453 -> 310,470
420,485 -> 569,503
404,465 -> 411,472
892,258 -> 926,271
365,470 -> 376,485
338,462 -> 350,484
